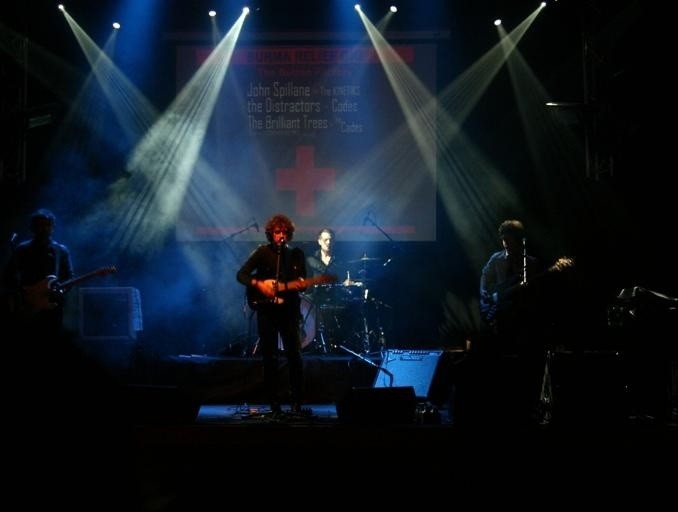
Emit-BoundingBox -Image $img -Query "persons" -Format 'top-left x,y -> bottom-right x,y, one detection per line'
1,209 -> 74,390
237,216 -> 322,402
479,217 -> 550,324
306,226 -> 355,342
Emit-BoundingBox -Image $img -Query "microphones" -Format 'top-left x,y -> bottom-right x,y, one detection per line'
362,211 -> 370,226
253,216 -> 260,232
280,239 -> 286,250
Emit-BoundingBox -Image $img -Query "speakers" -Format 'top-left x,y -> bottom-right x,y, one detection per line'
76,286 -> 144,340
537,350 -> 631,425
372,348 -> 454,403
334,386 -> 417,421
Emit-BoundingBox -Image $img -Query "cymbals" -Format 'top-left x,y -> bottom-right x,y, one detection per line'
349,257 -> 381,264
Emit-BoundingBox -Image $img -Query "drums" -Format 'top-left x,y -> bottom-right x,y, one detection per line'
342,279 -> 364,303
312,282 -> 347,308
277,292 -> 323,353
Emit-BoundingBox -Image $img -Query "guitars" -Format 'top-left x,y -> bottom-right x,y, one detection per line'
480,257 -> 575,329
25,266 -> 117,314
246,275 -> 337,311
